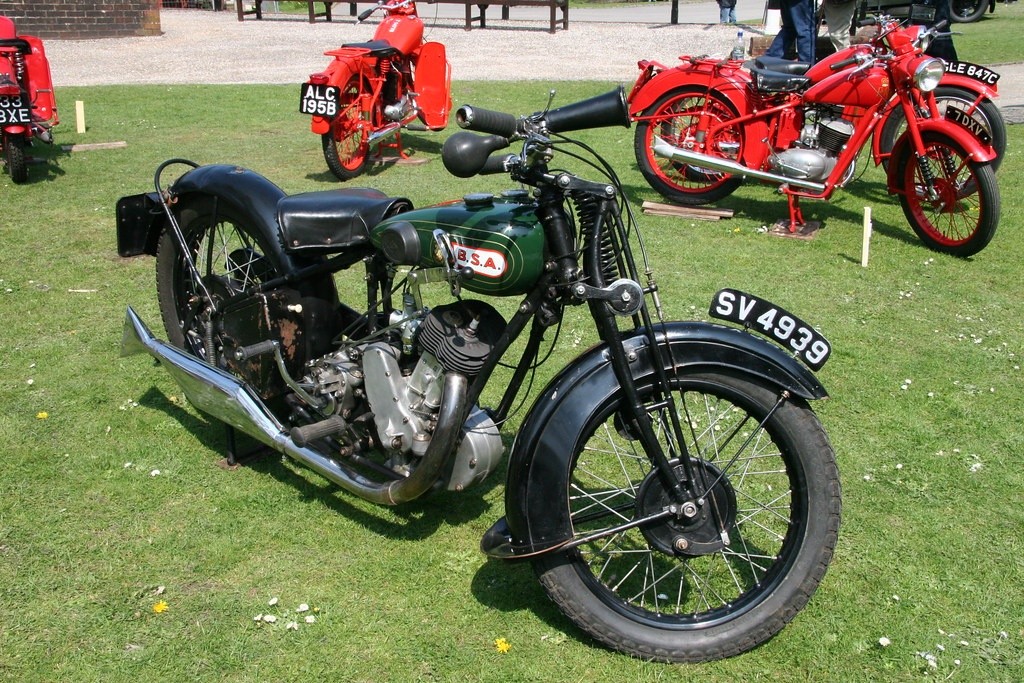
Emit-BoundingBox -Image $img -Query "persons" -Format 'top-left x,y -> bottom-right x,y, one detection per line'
815,0 -> 858,52
716,0 -> 737,23
764,0 -> 817,67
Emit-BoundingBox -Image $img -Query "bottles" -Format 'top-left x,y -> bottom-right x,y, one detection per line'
733,30 -> 744,61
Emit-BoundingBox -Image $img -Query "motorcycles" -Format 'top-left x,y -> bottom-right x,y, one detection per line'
660,13 -> 1008,202
854,0 -> 997,27
114,84 -> 842,664
0,15 -> 61,186
625,15 -> 1002,258
299,0 -> 453,181
